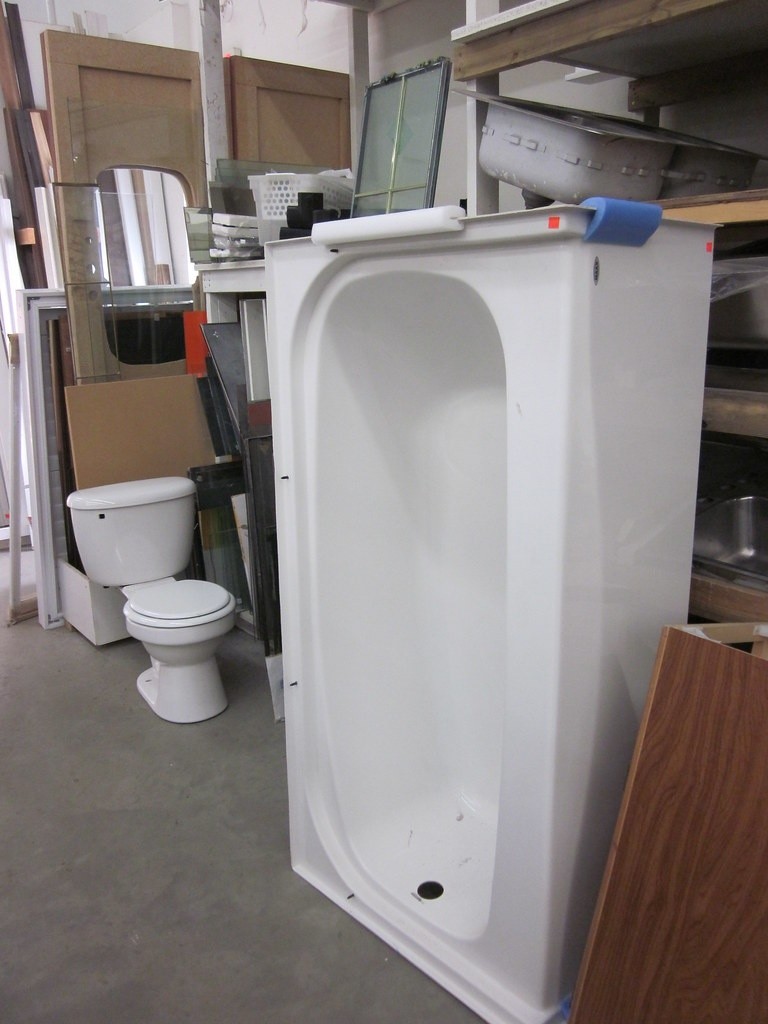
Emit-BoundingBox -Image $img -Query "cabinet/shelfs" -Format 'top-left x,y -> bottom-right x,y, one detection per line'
450,0 -> 768,622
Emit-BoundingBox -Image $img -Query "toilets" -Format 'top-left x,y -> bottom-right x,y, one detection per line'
64,474 -> 237,725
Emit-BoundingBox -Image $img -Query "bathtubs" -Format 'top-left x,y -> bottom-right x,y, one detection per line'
261,188 -> 721,1024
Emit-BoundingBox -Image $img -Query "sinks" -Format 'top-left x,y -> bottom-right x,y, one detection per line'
578,106 -> 768,203
447,82 -> 677,206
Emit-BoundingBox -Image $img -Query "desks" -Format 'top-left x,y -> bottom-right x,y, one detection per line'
195,259 -> 265,324
